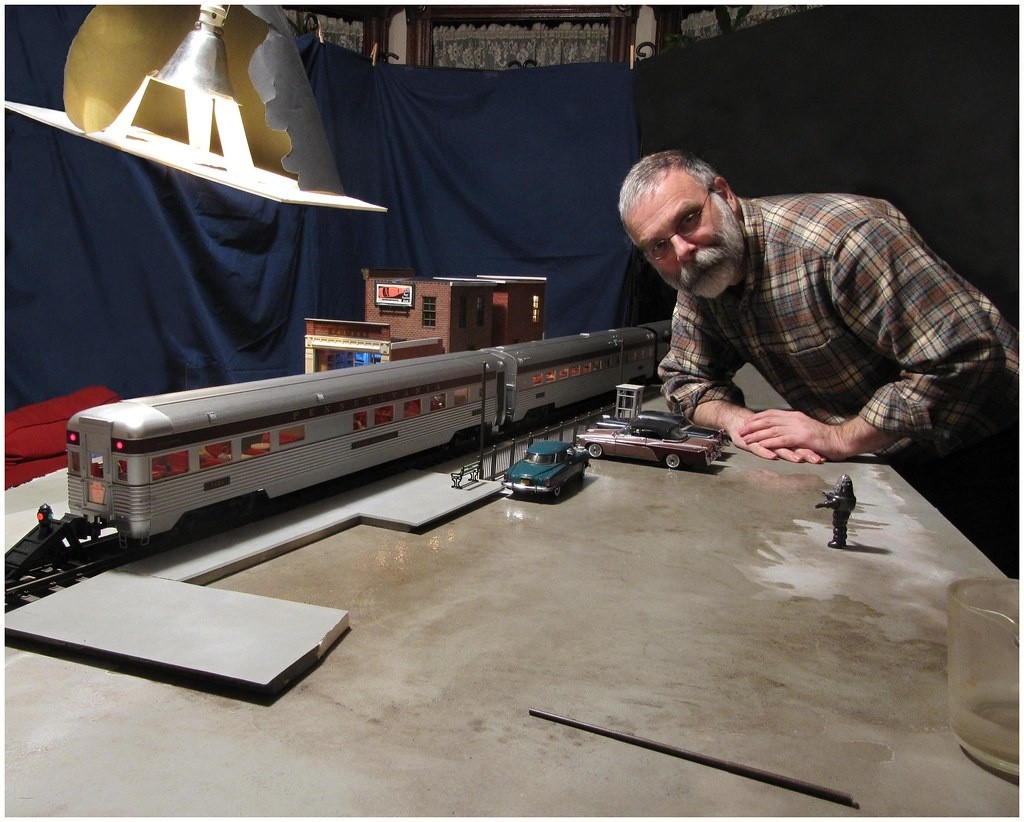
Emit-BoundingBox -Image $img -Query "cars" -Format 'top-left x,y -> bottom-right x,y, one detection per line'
501,440 -> 591,499
575,410 -> 731,468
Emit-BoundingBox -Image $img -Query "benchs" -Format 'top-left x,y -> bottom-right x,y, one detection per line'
451,460 -> 481,490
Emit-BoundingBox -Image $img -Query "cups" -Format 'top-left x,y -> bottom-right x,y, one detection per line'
948,576 -> 1019,776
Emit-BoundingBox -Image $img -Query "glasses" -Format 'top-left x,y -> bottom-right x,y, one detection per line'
644,189 -> 712,260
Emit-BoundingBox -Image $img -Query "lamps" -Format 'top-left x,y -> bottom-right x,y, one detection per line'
4,5 -> 387,212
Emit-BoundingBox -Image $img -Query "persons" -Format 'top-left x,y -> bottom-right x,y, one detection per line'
618,149 -> 1019,578
815,474 -> 856,548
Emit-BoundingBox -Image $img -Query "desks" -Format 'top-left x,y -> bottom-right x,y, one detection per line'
5,364 -> 1019,817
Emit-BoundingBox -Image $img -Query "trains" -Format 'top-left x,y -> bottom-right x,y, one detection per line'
65,319 -> 672,549
